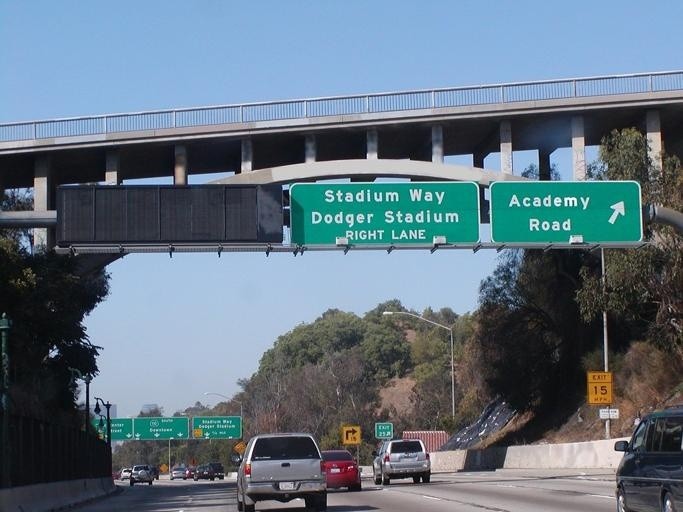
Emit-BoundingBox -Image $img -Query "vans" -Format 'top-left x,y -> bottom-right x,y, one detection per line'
612,403 -> 682,511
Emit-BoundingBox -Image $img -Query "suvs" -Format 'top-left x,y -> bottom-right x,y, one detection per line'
234,431 -> 328,512
372,438 -> 430,486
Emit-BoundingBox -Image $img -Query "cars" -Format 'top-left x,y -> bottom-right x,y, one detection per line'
111,460 -> 223,486
321,448 -> 362,492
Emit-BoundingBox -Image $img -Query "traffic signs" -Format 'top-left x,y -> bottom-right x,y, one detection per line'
586,372 -> 612,405
489,179 -> 644,247
191,415 -> 242,439
91,418 -> 132,440
374,422 -> 393,439
132,416 -> 189,441
287,184 -> 481,247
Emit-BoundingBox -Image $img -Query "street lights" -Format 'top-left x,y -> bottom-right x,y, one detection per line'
202,392 -> 242,444
66,365 -> 91,470
92,396 -> 111,445
168,437 -> 174,478
380,309 -> 455,422
